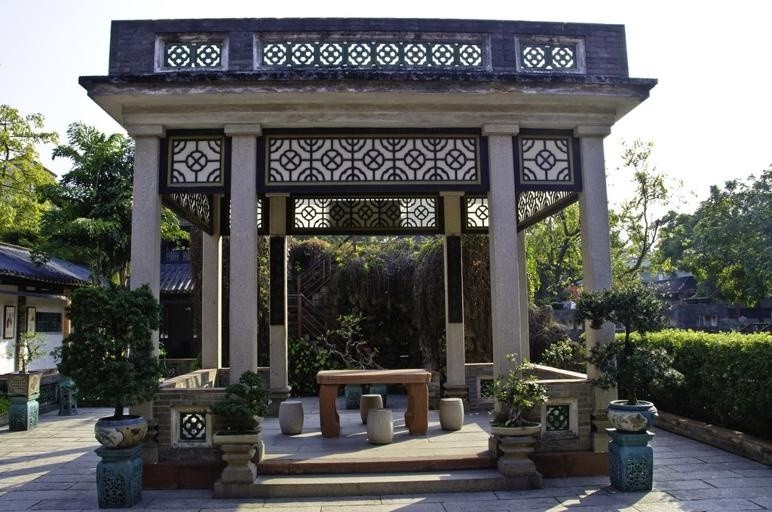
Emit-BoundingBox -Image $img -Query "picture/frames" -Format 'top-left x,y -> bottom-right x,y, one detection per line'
2,304 -> 17,340
25,306 -> 36,338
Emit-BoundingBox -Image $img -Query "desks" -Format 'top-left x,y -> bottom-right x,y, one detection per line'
315,367 -> 433,438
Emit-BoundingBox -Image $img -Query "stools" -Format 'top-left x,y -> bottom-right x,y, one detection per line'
278,399 -> 304,436
361,393 -> 385,426
439,396 -> 465,434
366,407 -> 394,445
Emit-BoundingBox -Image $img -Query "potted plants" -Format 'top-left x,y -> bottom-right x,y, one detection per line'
2,329 -> 48,397
208,367 -> 273,431
57,278 -> 168,449
314,309 -> 390,411
575,282 -> 672,432
481,350 -> 550,437
355,338 -> 388,408
